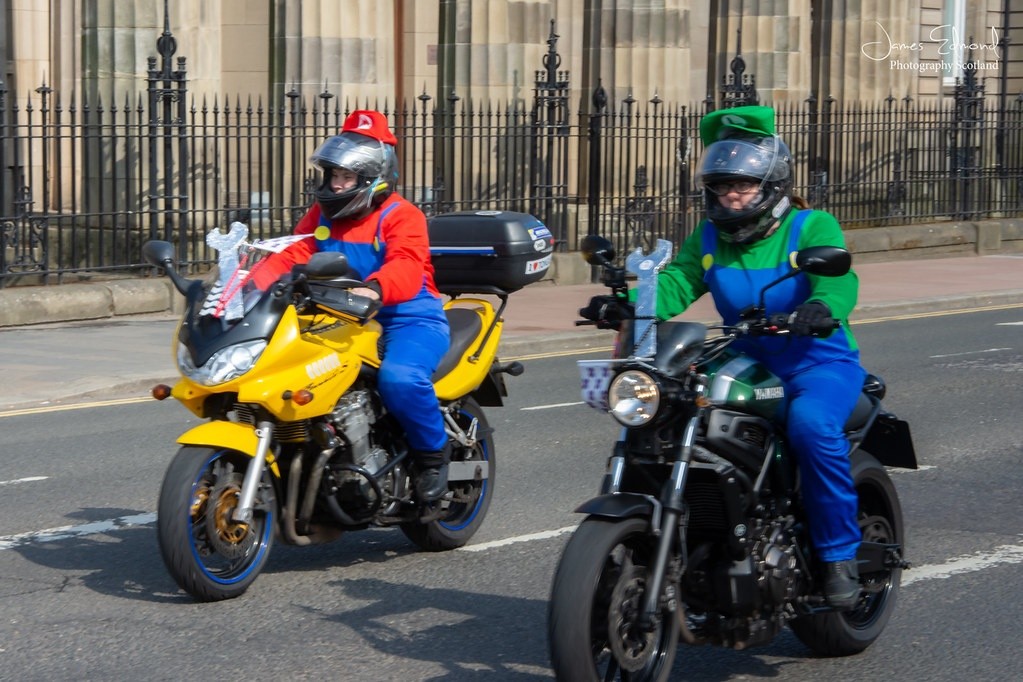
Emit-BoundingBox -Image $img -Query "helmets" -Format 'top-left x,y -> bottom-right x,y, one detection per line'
695,135 -> 795,246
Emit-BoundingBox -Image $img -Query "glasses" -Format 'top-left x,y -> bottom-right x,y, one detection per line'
712,181 -> 760,197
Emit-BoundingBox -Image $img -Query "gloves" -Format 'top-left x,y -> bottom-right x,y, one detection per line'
788,302 -> 830,338
587,294 -> 634,331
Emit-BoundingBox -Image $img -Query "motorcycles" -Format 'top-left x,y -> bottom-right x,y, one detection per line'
542,233 -> 922,682
137,209 -> 557,603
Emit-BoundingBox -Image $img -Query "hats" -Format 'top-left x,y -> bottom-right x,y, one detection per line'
311,132 -> 399,220
341,109 -> 398,146
701,105 -> 775,147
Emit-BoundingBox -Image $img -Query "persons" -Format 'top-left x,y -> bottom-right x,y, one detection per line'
586,106 -> 869,606
209,110 -> 452,503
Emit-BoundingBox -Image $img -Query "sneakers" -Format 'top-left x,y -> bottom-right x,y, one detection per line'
825,557 -> 860,606
412,438 -> 450,504
